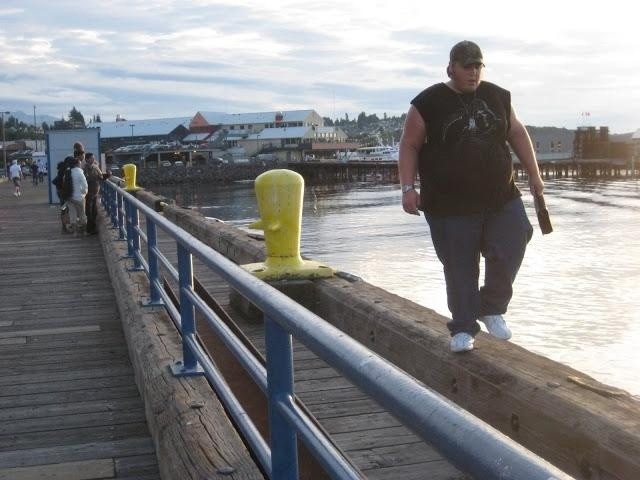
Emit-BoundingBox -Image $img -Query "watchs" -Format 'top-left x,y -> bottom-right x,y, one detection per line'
401,183 -> 416,194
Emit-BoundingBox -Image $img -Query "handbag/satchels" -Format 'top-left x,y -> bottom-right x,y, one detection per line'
61,176 -> 72,197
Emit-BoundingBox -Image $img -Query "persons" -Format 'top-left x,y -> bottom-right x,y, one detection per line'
37,159 -> 45,183
30,161 -> 40,187
398,39 -> 545,353
51,142 -> 112,238
9,159 -> 23,198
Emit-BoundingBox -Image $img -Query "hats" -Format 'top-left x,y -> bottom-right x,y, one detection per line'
450,41 -> 486,67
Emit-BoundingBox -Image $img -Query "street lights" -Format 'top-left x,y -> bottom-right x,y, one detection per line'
0,111 -> 10,178
34,107 -> 38,151
129,124 -> 136,144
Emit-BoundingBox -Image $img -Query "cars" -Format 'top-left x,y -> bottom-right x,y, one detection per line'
110,165 -> 120,170
212,157 -> 229,168
254,152 -> 280,167
160,160 -> 185,168
22,164 -> 49,178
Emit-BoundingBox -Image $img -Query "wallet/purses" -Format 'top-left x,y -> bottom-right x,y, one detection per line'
534,194 -> 553,234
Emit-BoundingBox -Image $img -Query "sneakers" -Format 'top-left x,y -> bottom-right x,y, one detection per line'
477,313 -> 511,339
14,192 -> 20,197
451,332 -> 474,353
